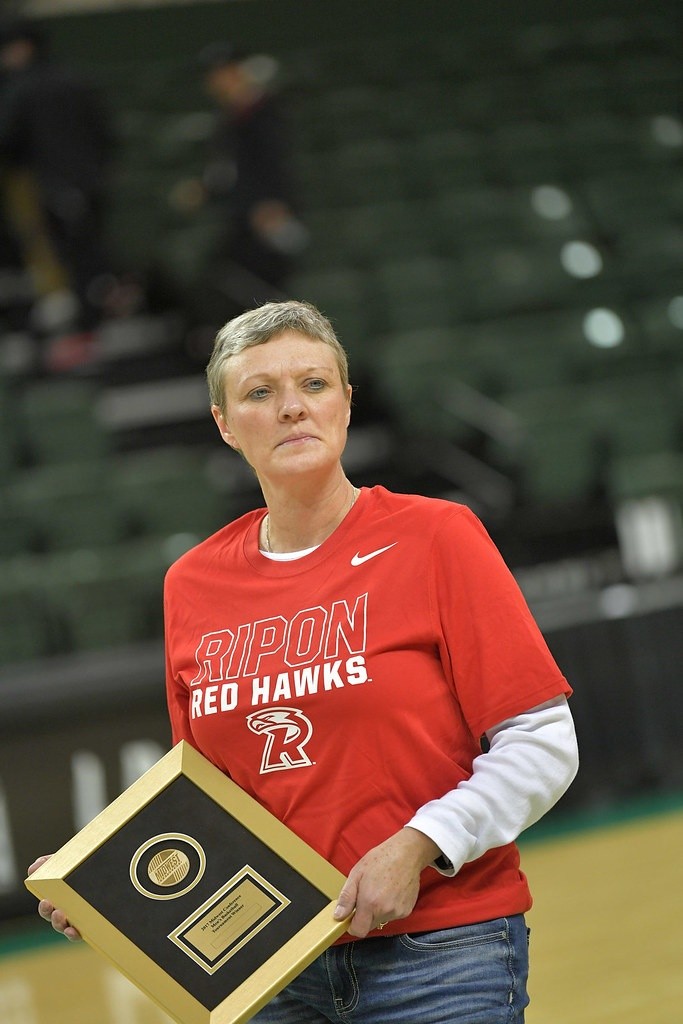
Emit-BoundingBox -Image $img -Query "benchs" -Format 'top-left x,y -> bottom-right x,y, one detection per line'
1,0 -> 682,665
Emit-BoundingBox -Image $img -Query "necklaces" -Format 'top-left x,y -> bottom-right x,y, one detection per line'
267,487 -> 356,553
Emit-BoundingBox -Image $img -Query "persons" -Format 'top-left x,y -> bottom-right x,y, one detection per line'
28,299 -> 582,1023
